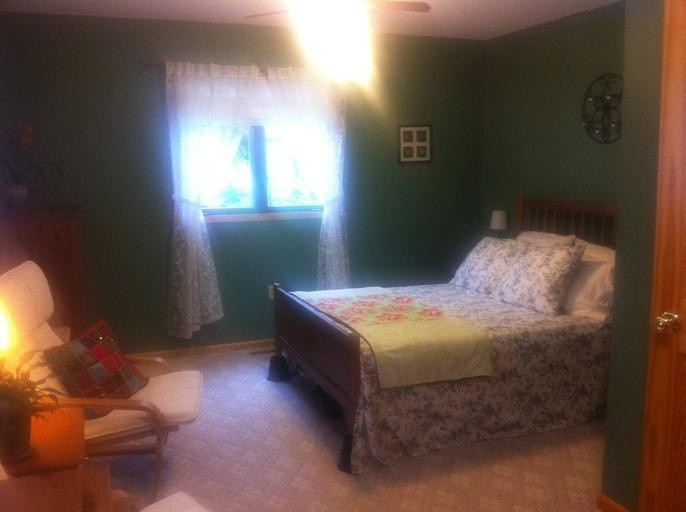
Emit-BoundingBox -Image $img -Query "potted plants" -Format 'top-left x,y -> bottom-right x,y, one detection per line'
2,154 -> 61,215
0,353 -> 67,464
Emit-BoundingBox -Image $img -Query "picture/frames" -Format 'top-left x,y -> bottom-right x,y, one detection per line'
398,124 -> 432,163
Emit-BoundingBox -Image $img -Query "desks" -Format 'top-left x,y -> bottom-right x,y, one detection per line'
0,404 -> 86,511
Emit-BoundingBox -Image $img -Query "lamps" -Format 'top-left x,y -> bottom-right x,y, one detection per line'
488,209 -> 509,238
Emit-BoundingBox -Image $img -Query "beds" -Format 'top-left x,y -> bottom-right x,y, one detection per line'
266,193 -> 619,475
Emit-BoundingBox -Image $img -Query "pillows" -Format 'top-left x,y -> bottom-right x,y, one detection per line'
43,318 -> 148,419
451,230 -> 615,318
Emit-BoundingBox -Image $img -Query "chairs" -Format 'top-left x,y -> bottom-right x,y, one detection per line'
0,260 -> 204,502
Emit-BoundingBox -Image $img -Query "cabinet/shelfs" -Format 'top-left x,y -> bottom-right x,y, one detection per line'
0,197 -> 87,341
